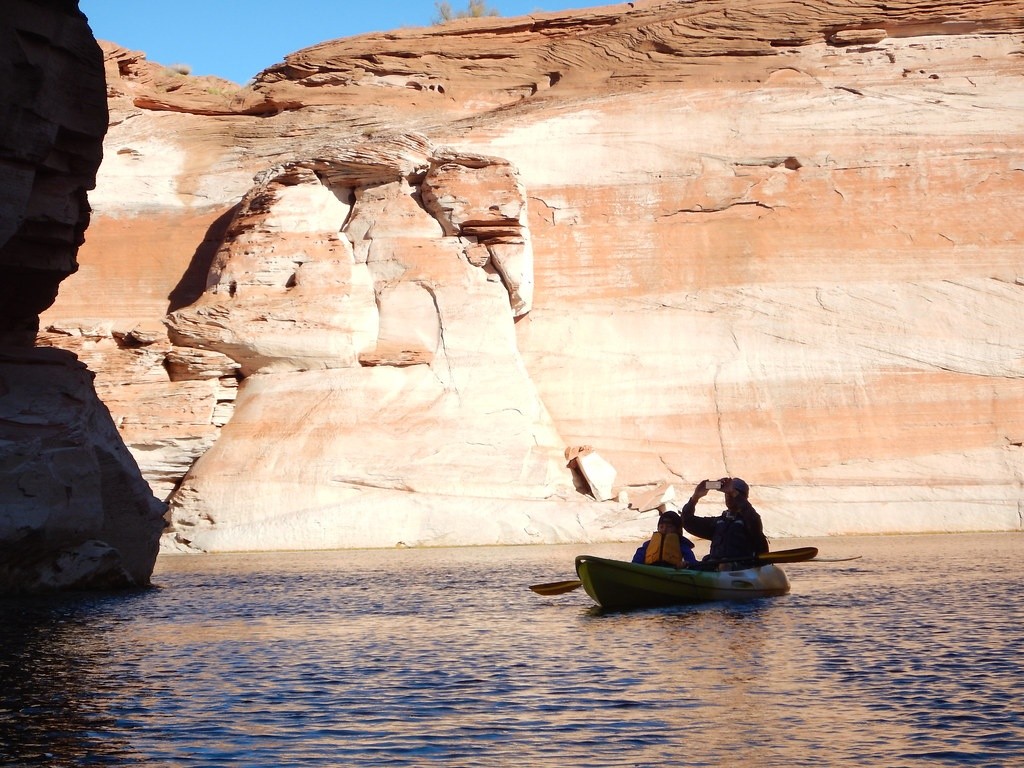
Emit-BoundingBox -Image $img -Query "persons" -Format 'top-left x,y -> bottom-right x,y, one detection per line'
681,477 -> 762,572
631,511 -> 698,570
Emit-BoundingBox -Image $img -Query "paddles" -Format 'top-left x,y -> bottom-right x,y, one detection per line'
529,546 -> 816,597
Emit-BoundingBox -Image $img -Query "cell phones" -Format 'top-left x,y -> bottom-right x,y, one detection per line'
706,481 -> 722,489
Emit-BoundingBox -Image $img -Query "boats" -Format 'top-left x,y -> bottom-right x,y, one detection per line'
574,555 -> 790,611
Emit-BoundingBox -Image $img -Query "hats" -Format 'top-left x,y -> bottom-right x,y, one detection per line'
733,477 -> 749,496
658,511 -> 681,530
